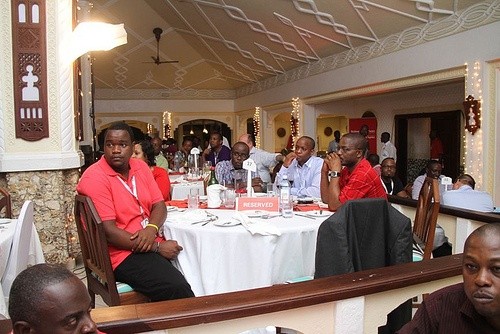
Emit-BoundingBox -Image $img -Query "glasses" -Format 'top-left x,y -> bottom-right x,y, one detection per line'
232,152 -> 250,159
381,164 -> 396,169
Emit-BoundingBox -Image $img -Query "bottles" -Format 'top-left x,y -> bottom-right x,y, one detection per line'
272,172 -> 291,208
207,166 -> 219,187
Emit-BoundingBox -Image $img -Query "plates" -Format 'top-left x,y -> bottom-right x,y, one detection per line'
213,219 -> 241,227
307,211 -> 331,217
0,219 -> 11,223
166,206 -> 178,212
242,210 -> 269,217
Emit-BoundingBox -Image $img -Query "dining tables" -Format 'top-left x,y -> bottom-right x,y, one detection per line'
157,192 -> 335,299
167,172 -> 212,201
0,218 -> 47,323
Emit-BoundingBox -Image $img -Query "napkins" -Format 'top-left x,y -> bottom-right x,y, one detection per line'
176,176 -> 190,185
167,208 -> 207,224
232,213 -> 281,236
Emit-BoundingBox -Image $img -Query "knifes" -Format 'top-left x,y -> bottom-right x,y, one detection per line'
192,220 -> 210,224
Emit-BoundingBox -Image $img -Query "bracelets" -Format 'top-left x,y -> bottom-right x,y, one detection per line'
146,224 -> 158,233
155,238 -> 163,254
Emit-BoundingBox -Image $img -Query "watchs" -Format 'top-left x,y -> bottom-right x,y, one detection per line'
329,171 -> 341,178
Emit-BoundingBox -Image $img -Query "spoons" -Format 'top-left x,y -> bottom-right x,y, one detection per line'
201,216 -> 218,226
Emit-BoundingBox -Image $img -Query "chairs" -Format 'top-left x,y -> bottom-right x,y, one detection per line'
0,201 -> 33,321
74,195 -> 150,307
412,178 -> 440,262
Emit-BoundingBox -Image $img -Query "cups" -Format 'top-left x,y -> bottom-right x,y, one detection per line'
187,188 -> 199,210
282,195 -> 293,217
224,188 -> 241,208
173,151 -> 212,181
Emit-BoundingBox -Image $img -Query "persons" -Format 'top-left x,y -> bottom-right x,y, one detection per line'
131,136 -> 171,202
215,129 -> 495,213
398,224 -> 500,334
150,124 -> 232,173
77,122 -> 196,303
7,263 -> 107,334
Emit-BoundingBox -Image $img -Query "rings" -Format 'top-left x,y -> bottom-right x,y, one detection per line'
329,157 -> 332,159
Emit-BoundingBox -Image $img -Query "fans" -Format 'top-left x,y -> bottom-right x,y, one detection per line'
144,27 -> 180,66
